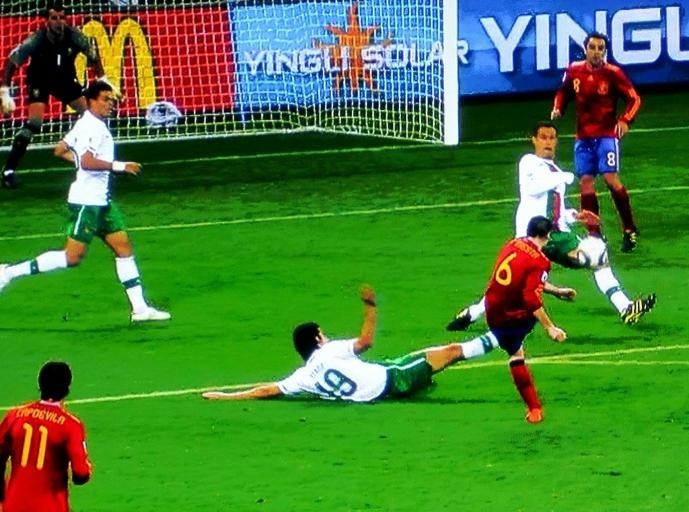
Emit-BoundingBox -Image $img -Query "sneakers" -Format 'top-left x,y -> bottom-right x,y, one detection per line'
2,265 -> 12,292
131,306 -> 171,321
620,294 -> 656,326
621,230 -> 640,253
2,170 -> 21,190
524,407 -> 544,422
446,309 -> 472,332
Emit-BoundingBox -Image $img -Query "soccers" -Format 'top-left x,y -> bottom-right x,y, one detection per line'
578,235 -> 608,267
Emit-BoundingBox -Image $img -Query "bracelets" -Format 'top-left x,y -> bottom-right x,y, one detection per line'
112,160 -> 127,174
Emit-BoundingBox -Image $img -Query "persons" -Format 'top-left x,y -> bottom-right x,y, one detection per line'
444,123 -> 657,330
0,361 -> 90,511
551,32 -> 642,252
202,286 -> 498,402
485,217 -> 576,422
0,81 -> 172,322
0,5 -> 117,189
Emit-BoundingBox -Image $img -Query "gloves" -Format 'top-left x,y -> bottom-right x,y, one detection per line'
0,86 -> 16,113
